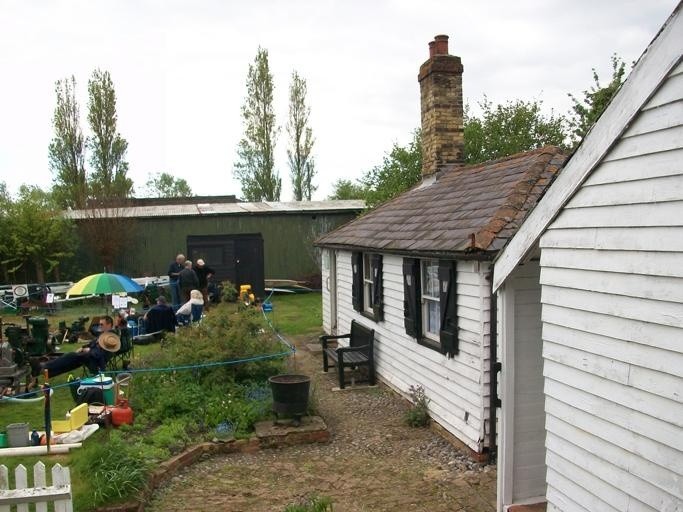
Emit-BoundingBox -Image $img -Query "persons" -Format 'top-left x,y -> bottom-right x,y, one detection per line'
142,296 -> 171,334
193,259 -> 215,314
174,289 -> 204,317
168,254 -> 185,305
28,314 -> 118,378
176,260 -> 207,318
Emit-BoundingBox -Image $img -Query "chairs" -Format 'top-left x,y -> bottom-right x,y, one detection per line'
77,303 -> 206,377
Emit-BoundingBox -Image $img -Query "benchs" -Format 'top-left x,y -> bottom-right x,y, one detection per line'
317,318 -> 376,390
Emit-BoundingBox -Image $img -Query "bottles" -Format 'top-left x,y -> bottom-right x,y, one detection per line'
31,429 -> 39,446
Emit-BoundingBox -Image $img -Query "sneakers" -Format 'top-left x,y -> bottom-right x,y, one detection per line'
31,360 -> 40,377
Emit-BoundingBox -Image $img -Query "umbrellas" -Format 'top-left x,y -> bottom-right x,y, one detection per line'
65,264 -> 145,317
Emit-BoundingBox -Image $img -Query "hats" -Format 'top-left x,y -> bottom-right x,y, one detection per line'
99,333 -> 122,352
155,295 -> 166,303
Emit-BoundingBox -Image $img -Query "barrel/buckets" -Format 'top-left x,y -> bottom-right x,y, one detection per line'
5,422 -> 31,447
